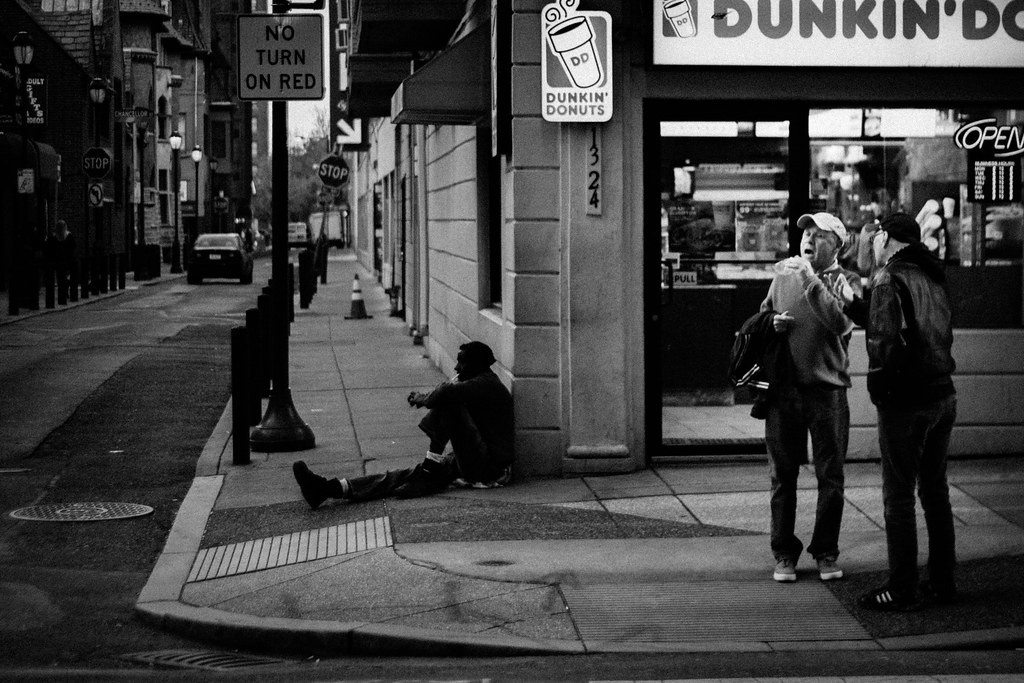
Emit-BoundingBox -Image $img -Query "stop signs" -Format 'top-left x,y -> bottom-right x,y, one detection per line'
317,155 -> 350,188
82,147 -> 115,180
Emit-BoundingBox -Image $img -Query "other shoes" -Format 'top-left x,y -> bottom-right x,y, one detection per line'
394,462 -> 436,500
293,459 -> 331,510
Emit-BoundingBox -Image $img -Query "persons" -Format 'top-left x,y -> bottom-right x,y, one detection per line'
292,340 -> 513,511
823,213 -> 957,610
49,219 -> 76,304
759,212 -> 864,581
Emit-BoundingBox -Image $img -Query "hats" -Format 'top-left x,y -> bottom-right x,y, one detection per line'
865,212 -> 921,244
796,212 -> 846,247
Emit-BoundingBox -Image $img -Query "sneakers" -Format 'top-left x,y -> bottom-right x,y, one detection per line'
858,585 -> 922,612
816,554 -> 842,580
773,555 -> 797,580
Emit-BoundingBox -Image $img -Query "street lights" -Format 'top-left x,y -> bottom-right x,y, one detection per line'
12,31 -> 39,311
191,144 -> 203,248
168,131 -> 183,273
88,77 -> 115,294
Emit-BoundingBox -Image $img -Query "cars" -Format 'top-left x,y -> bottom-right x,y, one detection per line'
186,233 -> 254,285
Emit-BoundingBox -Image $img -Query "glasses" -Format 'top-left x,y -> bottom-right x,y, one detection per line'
871,232 -> 884,243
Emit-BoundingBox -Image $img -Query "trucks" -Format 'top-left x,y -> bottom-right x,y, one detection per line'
288,211 -> 341,251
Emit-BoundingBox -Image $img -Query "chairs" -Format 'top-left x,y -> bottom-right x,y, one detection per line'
203,241 -> 210,247
226,241 -> 232,247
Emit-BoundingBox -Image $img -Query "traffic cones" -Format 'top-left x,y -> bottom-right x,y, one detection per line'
344,273 -> 373,319
